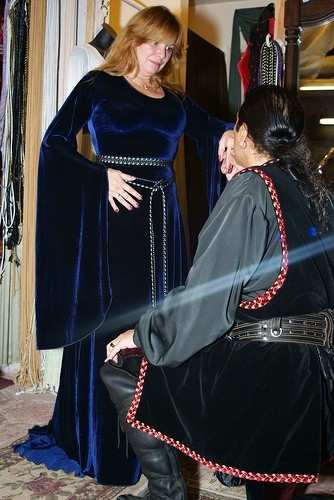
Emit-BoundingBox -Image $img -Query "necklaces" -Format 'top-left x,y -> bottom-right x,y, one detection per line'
128,76 -> 152,91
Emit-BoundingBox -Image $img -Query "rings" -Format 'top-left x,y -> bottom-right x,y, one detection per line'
115,193 -> 120,199
110,343 -> 116,347
227,147 -> 233,150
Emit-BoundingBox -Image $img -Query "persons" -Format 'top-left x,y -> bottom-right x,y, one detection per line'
100,84 -> 334,499
9,4 -> 235,486
65,22 -> 118,99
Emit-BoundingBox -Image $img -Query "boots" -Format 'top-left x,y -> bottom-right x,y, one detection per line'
98,354 -> 190,500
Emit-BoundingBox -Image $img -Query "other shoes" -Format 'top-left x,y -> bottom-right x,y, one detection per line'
214,471 -> 242,486
